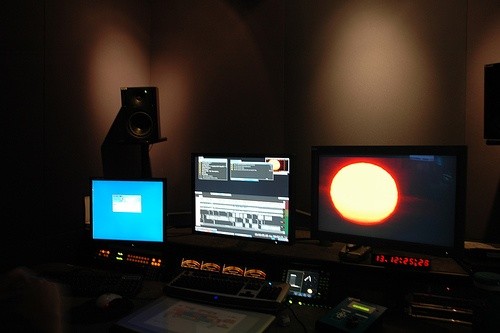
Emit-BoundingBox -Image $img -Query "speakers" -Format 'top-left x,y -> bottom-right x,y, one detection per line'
484,62 -> 500,140
127,87 -> 160,142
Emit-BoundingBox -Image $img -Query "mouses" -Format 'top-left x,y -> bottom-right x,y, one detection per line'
94,292 -> 133,315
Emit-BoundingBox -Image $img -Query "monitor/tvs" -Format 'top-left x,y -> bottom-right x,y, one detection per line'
89,176 -> 168,244
191,151 -> 296,246
310,145 -> 468,258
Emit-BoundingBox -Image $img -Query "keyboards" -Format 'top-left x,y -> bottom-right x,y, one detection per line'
90,247 -> 164,279
164,268 -> 290,313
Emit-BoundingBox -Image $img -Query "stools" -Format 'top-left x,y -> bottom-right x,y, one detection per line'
112,138 -> 164,178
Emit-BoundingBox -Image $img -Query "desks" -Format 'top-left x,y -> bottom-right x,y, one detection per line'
0,207 -> 500,333
